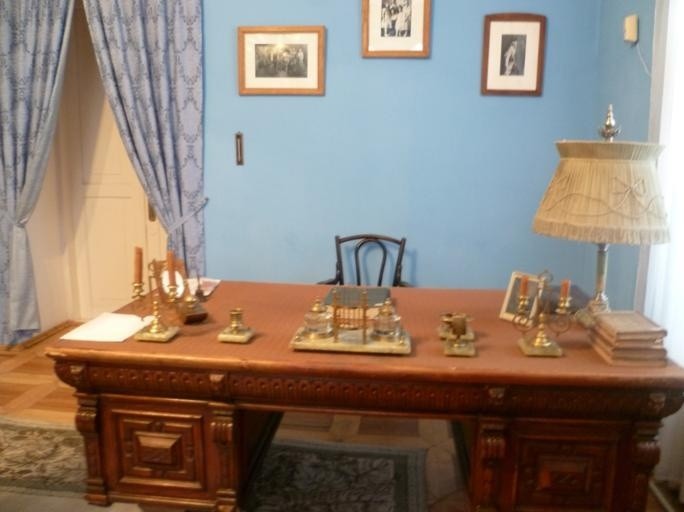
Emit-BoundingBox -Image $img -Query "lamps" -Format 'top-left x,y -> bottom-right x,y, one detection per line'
532,103 -> 671,328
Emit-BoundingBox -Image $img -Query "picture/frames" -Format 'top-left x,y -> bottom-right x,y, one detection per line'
361,0 -> 432,59
237,26 -> 326,94
499,272 -> 545,324
480,12 -> 547,96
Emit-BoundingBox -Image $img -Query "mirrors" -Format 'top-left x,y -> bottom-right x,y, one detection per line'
151,260 -> 190,302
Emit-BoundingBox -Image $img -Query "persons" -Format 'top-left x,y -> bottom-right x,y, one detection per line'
502,41 -> 518,75
381,0 -> 411,37
255,46 -> 307,77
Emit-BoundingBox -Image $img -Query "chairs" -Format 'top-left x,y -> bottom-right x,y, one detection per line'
313,234 -> 410,289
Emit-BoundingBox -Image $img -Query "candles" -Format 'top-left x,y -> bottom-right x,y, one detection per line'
520,277 -> 532,302
133,245 -> 142,280
561,278 -> 572,298
166,250 -> 177,281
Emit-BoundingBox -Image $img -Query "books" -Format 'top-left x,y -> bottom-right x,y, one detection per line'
587,310 -> 668,366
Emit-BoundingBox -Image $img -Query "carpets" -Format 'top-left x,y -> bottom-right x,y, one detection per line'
0,412 -> 431,512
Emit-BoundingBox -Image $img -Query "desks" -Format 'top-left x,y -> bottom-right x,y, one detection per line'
44,277 -> 683,511
